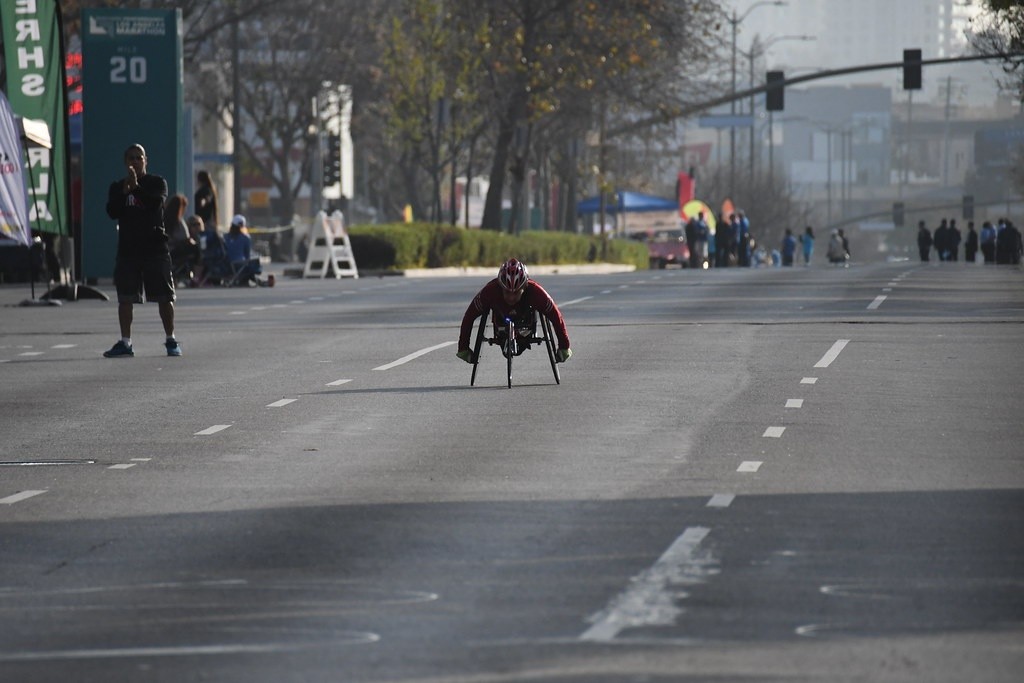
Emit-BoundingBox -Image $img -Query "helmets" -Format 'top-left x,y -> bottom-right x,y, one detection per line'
496,258 -> 529,293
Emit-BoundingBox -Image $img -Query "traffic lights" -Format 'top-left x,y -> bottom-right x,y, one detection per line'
322,130 -> 342,187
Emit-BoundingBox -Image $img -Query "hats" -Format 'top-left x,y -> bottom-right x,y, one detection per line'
231,214 -> 246,228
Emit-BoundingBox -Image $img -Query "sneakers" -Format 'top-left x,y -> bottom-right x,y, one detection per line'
163,338 -> 183,356
103,340 -> 135,358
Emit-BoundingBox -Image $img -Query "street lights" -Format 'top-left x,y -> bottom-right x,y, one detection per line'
707,0 -> 788,207
713,31 -> 859,233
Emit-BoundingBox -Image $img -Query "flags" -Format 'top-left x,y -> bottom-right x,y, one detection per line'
0,0 -> 74,239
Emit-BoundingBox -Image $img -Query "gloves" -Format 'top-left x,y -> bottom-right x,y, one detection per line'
455,346 -> 478,364
555,348 -> 573,364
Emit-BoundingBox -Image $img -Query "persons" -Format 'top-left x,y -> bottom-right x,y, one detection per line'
102,143 -> 182,358
980,218 -> 1024,264
917,221 -> 932,262
965,222 -> 978,262
799,227 -> 815,266
781,228 -> 797,267
164,170 -> 275,289
685,213 -> 783,269
947,220 -> 961,261
826,228 -> 851,268
934,219 -> 948,261
456,257 -> 572,364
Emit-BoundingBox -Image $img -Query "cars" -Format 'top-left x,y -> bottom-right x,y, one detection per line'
646,230 -> 691,270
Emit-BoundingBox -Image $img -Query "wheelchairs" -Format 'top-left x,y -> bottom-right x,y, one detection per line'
467,310 -> 562,389
